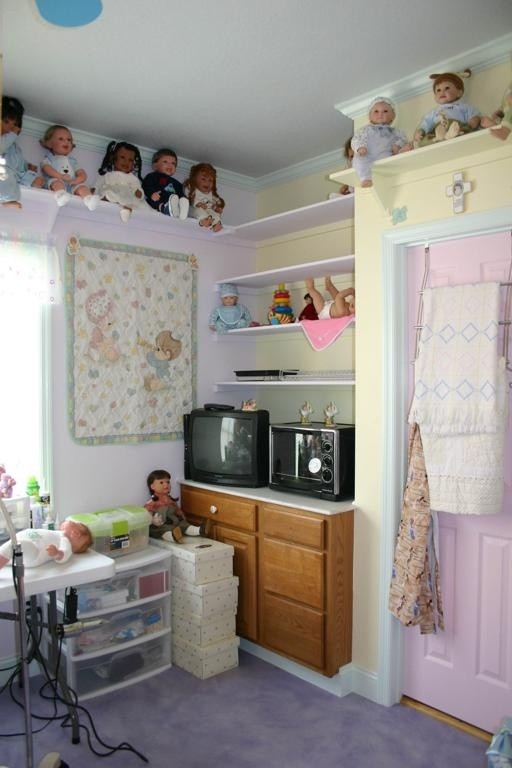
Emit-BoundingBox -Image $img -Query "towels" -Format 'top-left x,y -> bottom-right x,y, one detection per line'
407,282 -> 500,437
418,357 -> 510,515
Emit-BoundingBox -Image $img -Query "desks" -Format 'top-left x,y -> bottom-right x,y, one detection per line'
0,548 -> 115,744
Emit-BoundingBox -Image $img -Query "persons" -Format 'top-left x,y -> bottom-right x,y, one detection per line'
143,469 -> 214,545
0,520 -> 95,570
411,69 -> 481,147
350,96 -> 414,188
85,290 -> 117,361
298,293 -> 319,321
304,275 -> 355,319
208,283 -> 253,332
0,95 -> 226,233
143,330 -> 182,392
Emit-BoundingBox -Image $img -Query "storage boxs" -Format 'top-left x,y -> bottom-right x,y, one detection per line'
65,504 -> 152,559
171,633 -> 240,681
172,606 -> 237,648
169,577 -> 239,617
149,535 -> 235,586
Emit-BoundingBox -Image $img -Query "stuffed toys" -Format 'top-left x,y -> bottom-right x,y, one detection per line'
481,78 -> 512,140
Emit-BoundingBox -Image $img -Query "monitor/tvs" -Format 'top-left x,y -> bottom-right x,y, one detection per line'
189,408 -> 269,488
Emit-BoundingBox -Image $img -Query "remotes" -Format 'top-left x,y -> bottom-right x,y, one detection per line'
205,404 -> 234,411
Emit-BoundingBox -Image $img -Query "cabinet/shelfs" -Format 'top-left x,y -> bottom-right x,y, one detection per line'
180,485 -> 354,678
214,192 -> 355,387
35,546 -> 172,705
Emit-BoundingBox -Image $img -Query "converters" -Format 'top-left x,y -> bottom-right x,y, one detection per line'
66,595 -> 77,622
